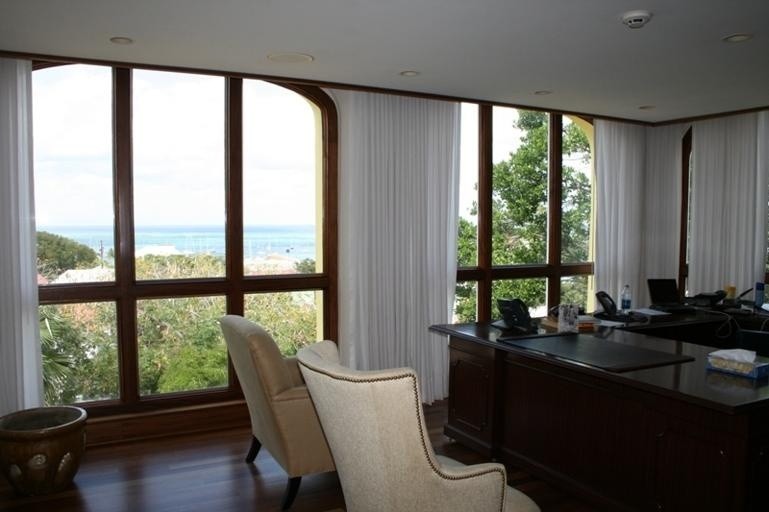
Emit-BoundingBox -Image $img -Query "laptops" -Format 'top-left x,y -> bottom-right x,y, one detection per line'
648,279 -> 696,313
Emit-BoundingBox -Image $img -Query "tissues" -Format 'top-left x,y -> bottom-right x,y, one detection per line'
704,349 -> 768,381
702,367 -> 769,399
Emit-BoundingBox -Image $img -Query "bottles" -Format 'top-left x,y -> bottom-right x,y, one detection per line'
620,284 -> 632,315
754,282 -> 765,307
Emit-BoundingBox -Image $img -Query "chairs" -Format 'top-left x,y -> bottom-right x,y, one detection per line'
220,315 -> 337,511
295,339 -> 542,512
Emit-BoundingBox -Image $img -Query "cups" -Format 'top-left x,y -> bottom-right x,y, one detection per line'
723,285 -> 736,298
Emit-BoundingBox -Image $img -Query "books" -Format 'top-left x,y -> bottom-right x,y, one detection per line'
540,317 -> 594,328
578,315 -> 602,323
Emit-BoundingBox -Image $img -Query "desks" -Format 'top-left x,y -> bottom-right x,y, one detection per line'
428,305 -> 769,512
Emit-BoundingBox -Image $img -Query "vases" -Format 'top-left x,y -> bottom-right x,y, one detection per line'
0,405 -> 88,498
